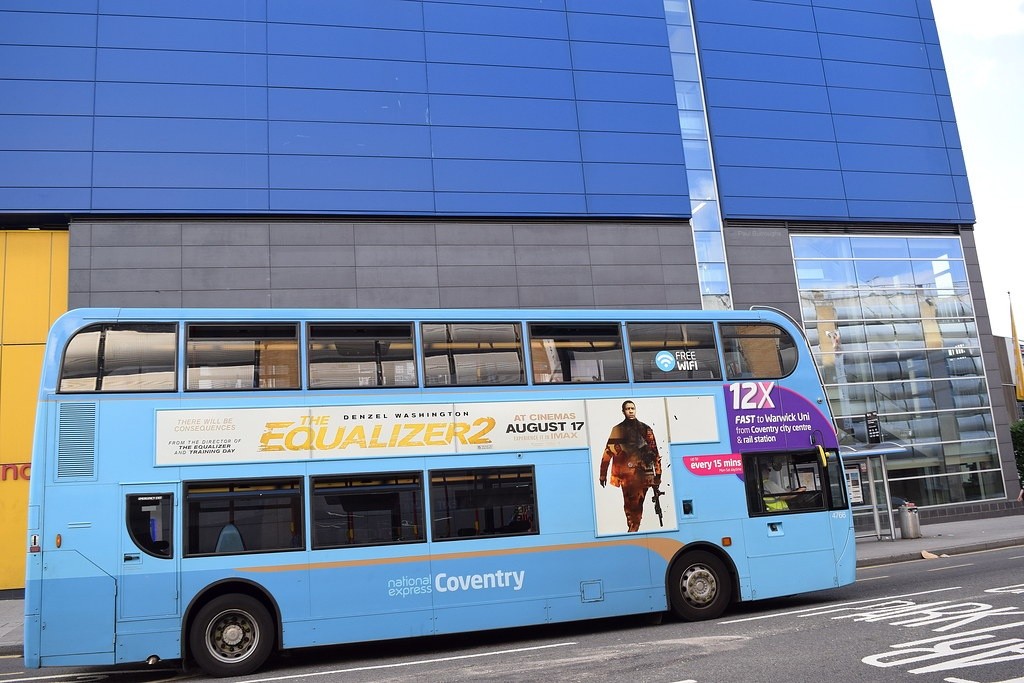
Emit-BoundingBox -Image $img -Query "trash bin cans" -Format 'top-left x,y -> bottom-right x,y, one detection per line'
897,506 -> 923,539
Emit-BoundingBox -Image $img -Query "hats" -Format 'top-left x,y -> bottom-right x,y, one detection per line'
771,462 -> 784,466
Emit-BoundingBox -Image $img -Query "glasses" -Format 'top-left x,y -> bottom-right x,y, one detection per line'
767,468 -> 771,472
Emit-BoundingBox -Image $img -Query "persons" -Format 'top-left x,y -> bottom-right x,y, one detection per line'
1016,485 -> 1024,503
600,400 -> 662,532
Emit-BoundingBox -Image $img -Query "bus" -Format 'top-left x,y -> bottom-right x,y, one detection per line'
23,305 -> 856,678
